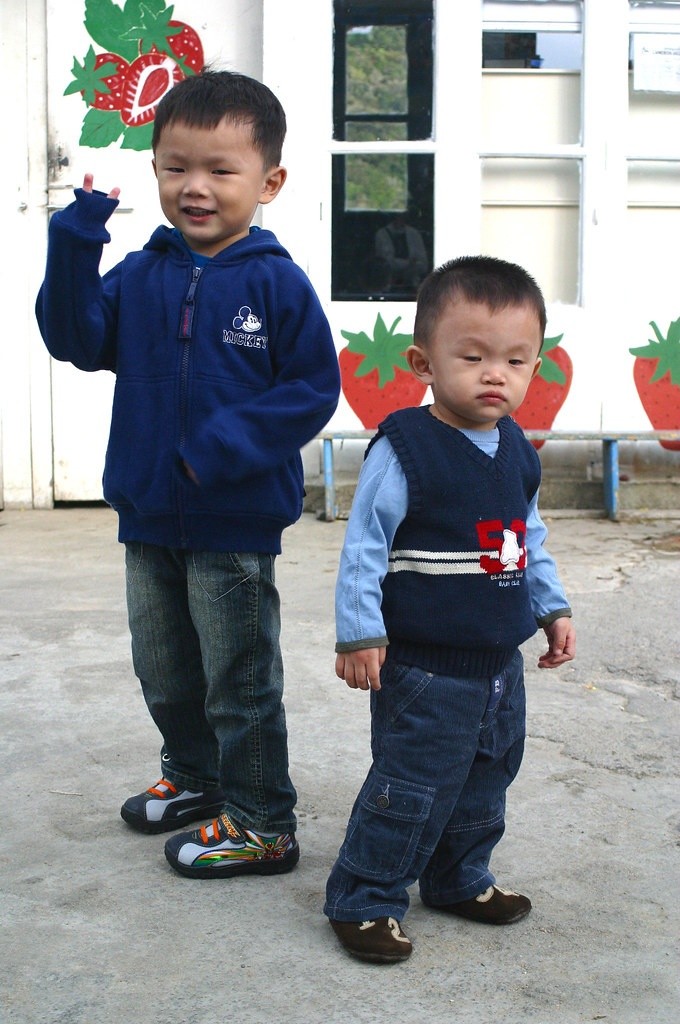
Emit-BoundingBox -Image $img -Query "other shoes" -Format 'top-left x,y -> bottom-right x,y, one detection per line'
436,885 -> 533,926
328,913 -> 412,961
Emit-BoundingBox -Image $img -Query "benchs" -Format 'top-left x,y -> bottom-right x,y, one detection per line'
315,429 -> 680,523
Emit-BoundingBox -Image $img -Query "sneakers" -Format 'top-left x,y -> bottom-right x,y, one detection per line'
120,776 -> 226,834
164,812 -> 300,879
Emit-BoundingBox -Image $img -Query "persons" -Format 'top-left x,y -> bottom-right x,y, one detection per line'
322,256 -> 576,962
36,70 -> 342,878
